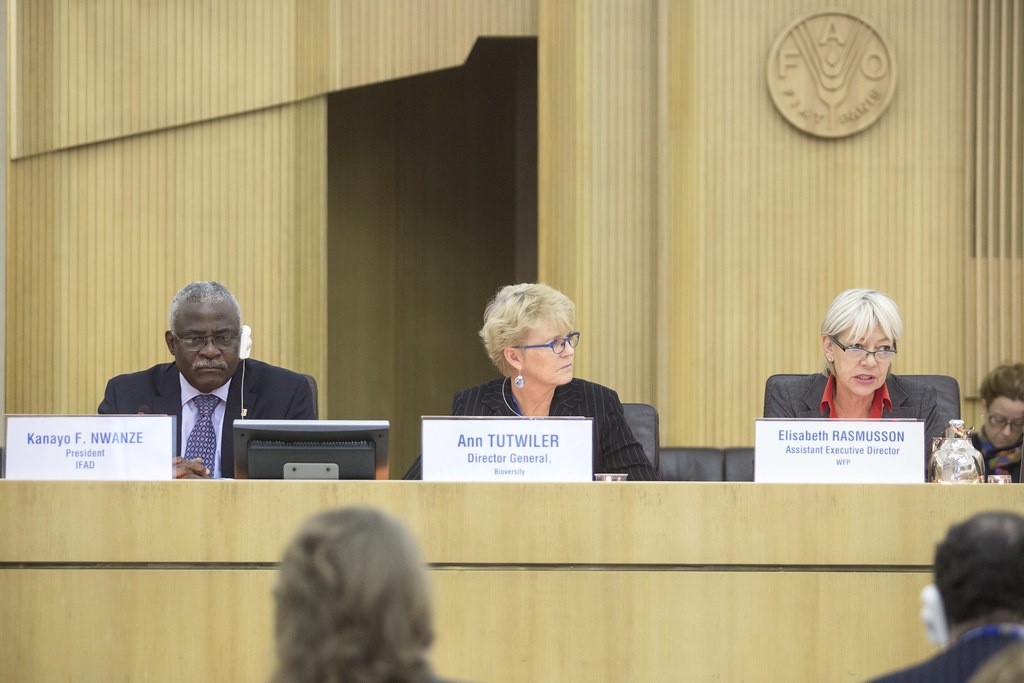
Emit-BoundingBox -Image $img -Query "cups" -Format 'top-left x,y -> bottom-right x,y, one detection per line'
987,474 -> 1012,483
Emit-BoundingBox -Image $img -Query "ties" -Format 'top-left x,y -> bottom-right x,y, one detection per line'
184,394 -> 221,479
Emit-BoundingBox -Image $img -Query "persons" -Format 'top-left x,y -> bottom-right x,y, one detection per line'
99,281 -> 315,479
866,510 -> 1024,683
266,506 -> 463,683
400,283 -> 658,481
765,288 -> 942,484
969,363 -> 1024,484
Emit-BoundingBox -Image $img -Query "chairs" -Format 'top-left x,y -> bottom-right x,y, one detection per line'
621,404 -> 659,480
764,374 -> 961,437
658,448 -> 754,481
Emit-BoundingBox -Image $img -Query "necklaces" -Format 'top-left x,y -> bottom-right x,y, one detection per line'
502,376 -> 522,418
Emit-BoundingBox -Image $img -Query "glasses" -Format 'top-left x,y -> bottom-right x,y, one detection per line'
987,403 -> 1024,432
829,335 -> 898,363
511,332 -> 580,355
172,329 -> 242,352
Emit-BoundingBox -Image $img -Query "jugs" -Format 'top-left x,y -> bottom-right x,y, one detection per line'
929,419 -> 986,484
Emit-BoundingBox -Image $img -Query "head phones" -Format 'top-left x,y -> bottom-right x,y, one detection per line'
921,584 -> 951,646
237,325 -> 252,359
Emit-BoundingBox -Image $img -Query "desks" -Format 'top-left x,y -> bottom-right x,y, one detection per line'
0,477 -> 1024,683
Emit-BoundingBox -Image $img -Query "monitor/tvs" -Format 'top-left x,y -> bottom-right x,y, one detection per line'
232,418 -> 390,482
